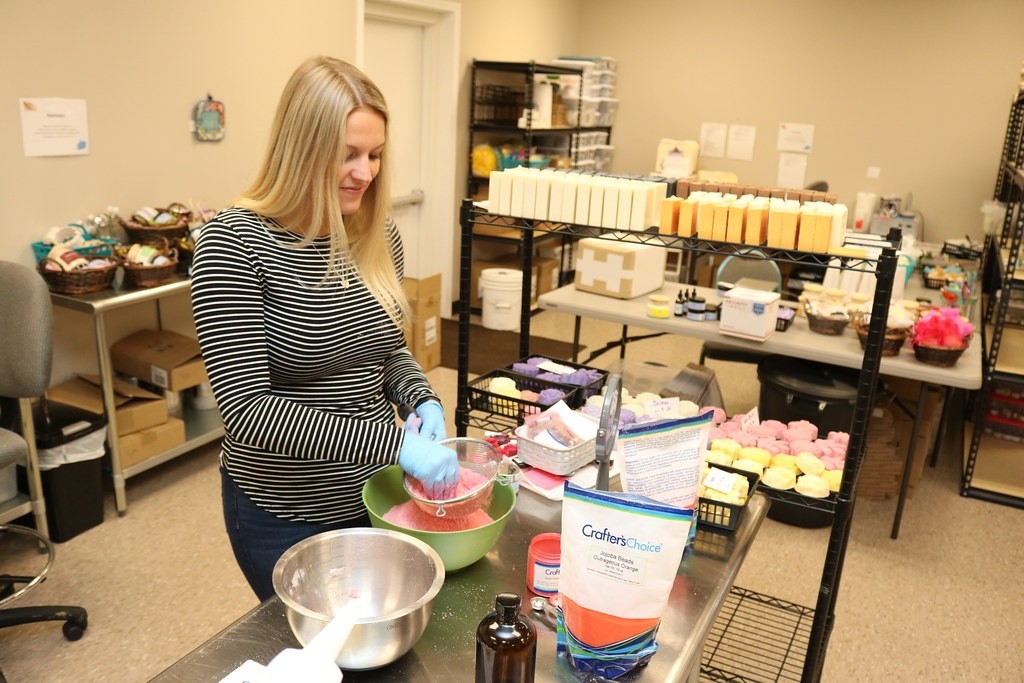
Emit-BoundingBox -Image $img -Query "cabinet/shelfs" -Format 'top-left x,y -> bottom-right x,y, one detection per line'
452,195 -> 903,683
959,101 -> 1024,510
46,272 -> 242,516
467,58 -> 584,207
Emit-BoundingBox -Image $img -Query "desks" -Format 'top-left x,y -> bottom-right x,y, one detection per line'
540,283 -> 981,539
146,453 -> 774,683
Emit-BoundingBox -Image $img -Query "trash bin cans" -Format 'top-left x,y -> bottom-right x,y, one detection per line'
14,405 -> 110,544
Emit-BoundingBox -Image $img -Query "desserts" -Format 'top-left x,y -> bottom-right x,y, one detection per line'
487,358 -> 603,416
584,382 -> 849,526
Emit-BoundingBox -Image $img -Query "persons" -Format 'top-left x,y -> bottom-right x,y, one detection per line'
191,54 -> 458,604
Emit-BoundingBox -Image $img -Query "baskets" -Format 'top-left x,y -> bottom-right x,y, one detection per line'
855,312 -> 913,357
804,299 -> 855,335
515,410 -> 601,475
919,255 -> 967,290
911,315 -> 974,368
466,370 -> 578,421
943,242 -> 984,261
696,461 -> 760,530
716,303 -> 798,332
504,353 -> 611,406
32,202 -> 218,294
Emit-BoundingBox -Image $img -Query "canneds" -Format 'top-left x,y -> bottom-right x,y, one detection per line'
526,533 -> 562,597
646,294 -> 672,319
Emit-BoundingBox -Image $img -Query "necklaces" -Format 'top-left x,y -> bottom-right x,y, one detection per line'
291,216 -> 349,288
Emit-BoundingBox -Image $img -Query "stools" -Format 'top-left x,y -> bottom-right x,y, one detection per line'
0,262 -> 89,683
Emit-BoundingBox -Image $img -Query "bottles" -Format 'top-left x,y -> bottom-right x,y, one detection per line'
475,592 -> 537,683
498,463 -> 520,493
526,533 -> 561,597
674,289 -> 718,321
647,294 -> 671,318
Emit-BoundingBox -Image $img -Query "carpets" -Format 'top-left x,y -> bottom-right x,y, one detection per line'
439,318 -> 585,378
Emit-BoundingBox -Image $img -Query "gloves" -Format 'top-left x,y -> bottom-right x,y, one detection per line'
403,402 -> 447,446
398,430 -> 459,501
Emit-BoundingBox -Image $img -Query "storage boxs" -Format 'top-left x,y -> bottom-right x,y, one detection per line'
574,234 -> 667,300
117,417 -> 186,469
108,329 -> 209,393
401,272 -> 443,376
44,375 -> 169,437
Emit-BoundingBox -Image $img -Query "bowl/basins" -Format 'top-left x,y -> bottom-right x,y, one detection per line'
272,527 -> 445,670
362,466 -> 516,570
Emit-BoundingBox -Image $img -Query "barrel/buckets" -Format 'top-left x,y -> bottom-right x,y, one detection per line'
482,268 -> 523,330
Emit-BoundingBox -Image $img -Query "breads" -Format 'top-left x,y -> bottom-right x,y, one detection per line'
488,166 -> 849,299
